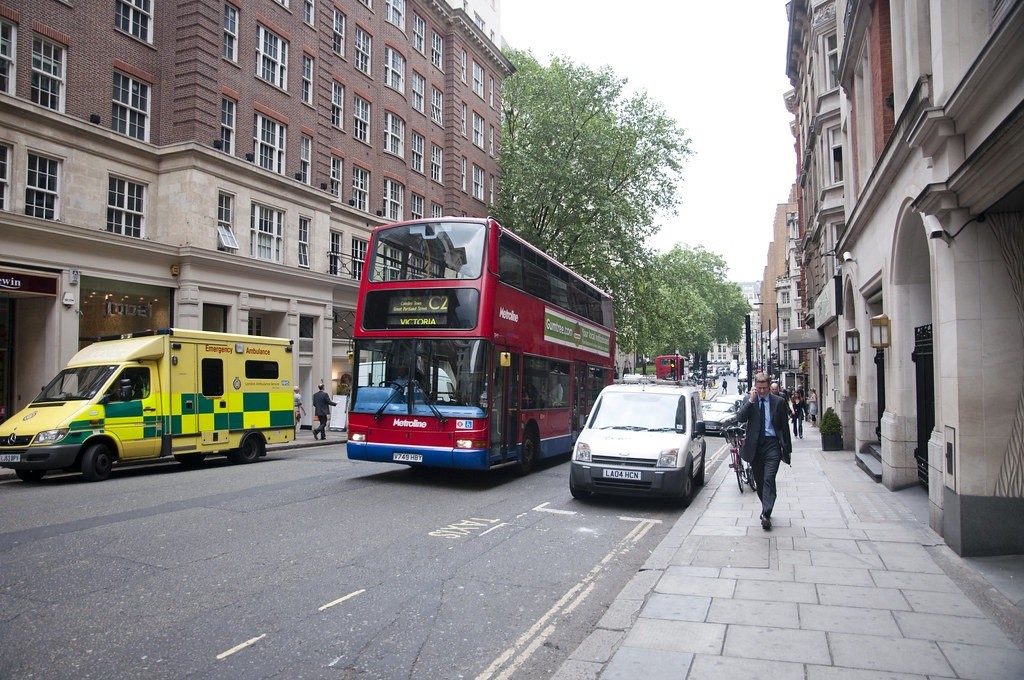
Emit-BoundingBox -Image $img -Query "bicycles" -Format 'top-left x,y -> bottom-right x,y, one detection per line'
719,419 -> 758,494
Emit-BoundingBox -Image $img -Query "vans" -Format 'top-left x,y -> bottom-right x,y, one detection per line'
569,383 -> 707,501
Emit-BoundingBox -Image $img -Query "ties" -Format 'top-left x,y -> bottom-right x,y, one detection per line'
759,397 -> 766,445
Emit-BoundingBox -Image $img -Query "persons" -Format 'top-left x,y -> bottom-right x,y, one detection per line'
293,385 -> 306,440
738,383 -> 742,395
737,373 -> 792,530
312,383 -> 341,440
614,359 -> 632,379
722,379 -> 727,395
543,372 -> 563,407
390,362 -> 414,395
770,377 -> 817,439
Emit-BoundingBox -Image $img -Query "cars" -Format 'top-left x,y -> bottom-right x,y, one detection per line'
707,365 -> 732,378
716,393 -> 746,410
700,402 -> 738,437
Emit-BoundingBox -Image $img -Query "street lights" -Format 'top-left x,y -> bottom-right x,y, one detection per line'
753,302 -> 781,391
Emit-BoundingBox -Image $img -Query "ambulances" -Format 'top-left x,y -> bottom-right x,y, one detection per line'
0,328 -> 296,481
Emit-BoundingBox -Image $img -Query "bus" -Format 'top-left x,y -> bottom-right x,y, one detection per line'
346,216 -> 617,473
656,356 -> 689,383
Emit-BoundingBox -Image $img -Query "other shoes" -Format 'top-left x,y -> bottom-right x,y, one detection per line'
313,432 -> 319,440
762,517 -> 771,529
321,436 -> 326,440
800,435 -> 802,439
795,435 -> 797,438
760,513 -> 764,519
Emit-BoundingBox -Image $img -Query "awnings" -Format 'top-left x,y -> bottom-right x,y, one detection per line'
788,329 -> 826,350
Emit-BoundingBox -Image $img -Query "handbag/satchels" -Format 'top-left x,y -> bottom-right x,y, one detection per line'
296,411 -> 301,419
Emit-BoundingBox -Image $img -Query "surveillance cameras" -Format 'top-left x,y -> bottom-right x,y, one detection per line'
924,215 -> 944,239
843,251 -> 852,261
